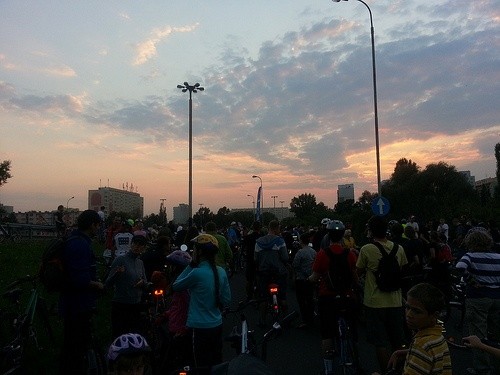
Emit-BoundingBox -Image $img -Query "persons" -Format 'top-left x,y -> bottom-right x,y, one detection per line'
45,204 -> 500,375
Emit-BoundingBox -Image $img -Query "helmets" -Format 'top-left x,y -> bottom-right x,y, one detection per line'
189,233 -> 220,257
131,231 -> 147,243
166,249 -> 193,267
106,334 -> 150,372
326,220 -> 345,232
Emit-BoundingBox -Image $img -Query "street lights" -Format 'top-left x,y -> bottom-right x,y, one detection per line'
177,82 -> 204,227
252,176 -> 264,225
331,0 -> 381,197
67,197 -> 74,209
279,201 -> 285,219
271,196 -> 278,210
160,199 -> 167,208
248,195 -> 255,212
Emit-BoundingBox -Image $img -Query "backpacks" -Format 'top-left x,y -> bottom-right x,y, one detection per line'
372,240 -> 404,292
324,246 -> 358,298
39,232 -> 90,295
